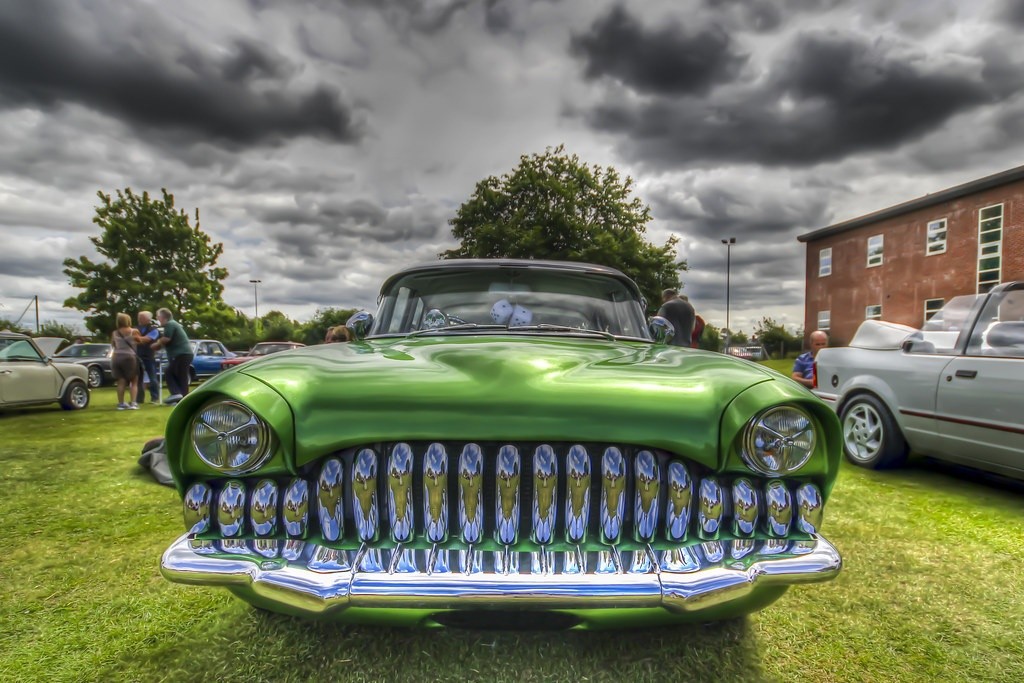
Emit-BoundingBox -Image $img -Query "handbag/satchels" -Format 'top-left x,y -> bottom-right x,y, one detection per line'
136,355 -> 146,371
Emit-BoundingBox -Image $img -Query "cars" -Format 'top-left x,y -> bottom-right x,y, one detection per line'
154,339 -> 243,381
723,347 -> 753,361
0,337 -> 69,363
151,261 -> 844,635
800,281 -> 1024,488
1,333 -> 90,412
54,342 -> 116,388
746,346 -> 764,359
222,341 -> 309,374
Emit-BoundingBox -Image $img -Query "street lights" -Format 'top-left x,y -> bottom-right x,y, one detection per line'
722,237 -> 737,354
249,280 -> 261,321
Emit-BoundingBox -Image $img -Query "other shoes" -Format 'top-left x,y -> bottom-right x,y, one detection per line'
117,403 -> 127,410
163,394 -> 183,405
150,397 -> 157,404
129,402 -> 140,410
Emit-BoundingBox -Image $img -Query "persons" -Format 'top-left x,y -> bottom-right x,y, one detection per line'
151,307 -> 193,407
111,311 -> 162,410
71,337 -> 92,357
654,290 -> 705,350
326,324 -> 350,344
792,330 -> 828,390
